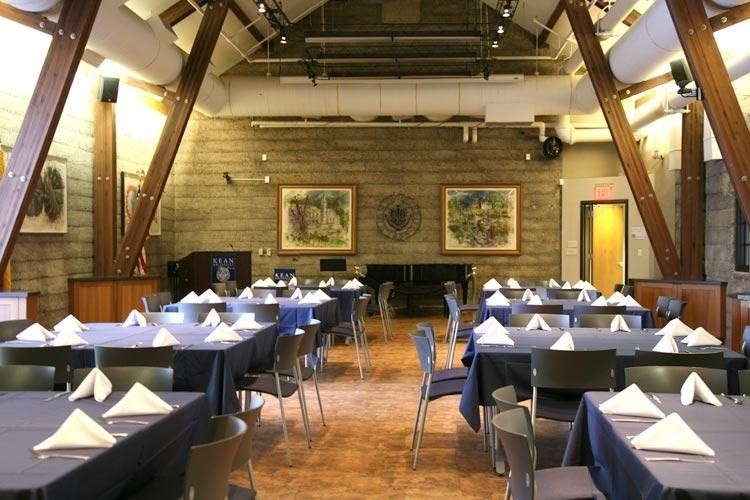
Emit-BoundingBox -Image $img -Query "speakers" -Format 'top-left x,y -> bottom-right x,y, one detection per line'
96,76 -> 120,104
669,59 -> 692,94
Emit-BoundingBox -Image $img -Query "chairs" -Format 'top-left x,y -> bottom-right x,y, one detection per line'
157,291 -> 173,311
234,303 -> 279,326
264,319 -> 326,440
179,304 -> 226,324
410,322 -> 496,472
318,294 -> 373,380
235,329 -> 311,467
573,305 -> 625,315
492,385 -> 530,500
143,295 -> 162,314
510,313 -> 570,330
93,346 -> 173,394
573,314 -> 644,329
511,305 -> 563,315
445,281 -> 480,335
366,281 -> 395,343
655,297 -> 687,326
221,397 -> 265,500
0,346 -> 70,393
624,366 -> 729,396
491,386 -> 603,500
130,416 -> 247,500
445,296 -> 473,370
530,350 -> 617,433
635,350 -> 725,369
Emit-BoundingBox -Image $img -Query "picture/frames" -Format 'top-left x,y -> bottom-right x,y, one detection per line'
277,185 -> 357,255
442,183 -> 522,256
122,172 -> 161,237
2,145 -> 69,236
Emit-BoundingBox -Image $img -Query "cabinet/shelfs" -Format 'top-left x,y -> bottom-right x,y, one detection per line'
68,277 -> 161,324
0,291 -> 40,322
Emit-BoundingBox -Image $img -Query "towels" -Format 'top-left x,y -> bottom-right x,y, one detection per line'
298,291 -> 322,304
610,314 -> 631,333
326,276 -> 335,287
473,314 -> 511,334
522,288 -> 534,302
238,286 -> 254,299
483,282 -> 503,291
341,279 -> 360,289
204,321 -> 242,343
524,312 -> 553,333
548,278 -> 562,288
352,277 -> 365,287
652,334 -> 680,354
485,290 -> 511,302
629,411 -> 716,457
616,292 -> 642,307
590,295 -> 608,307
607,290 -> 625,303
50,329 -> 89,347
475,326 -> 514,345
200,288 -> 223,303
122,308 -> 148,329
289,276 -> 298,286
230,313 -> 264,331
68,367 -> 113,403
654,317 -> 694,337
680,372 -> 722,408
584,280 -> 598,290
577,288 -> 591,303
102,381 -> 175,418
598,382 -> 665,419
549,331 -> 575,351
484,278 -> 502,287
276,279 -> 287,288
33,407 -> 118,451
15,322 -> 57,343
318,279 -> 327,287
53,313 -> 89,332
152,327 -> 183,347
507,277 -> 514,286
572,280 -> 589,289
487,300 -> 511,307
510,280 -> 521,289
527,294 -> 543,306
264,293 -> 279,304
251,278 -> 269,287
291,287 -> 303,300
181,290 -> 206,303
561,281 -> 572,290
314,288 -> 332,301
264,276 -> 277,287
202,308 -> 222,328
681,326 -> 723,347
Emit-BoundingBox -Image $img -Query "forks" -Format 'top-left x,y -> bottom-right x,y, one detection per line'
100,417 -> 149,425
29,450 -> 91,461
721,393 -> 743,405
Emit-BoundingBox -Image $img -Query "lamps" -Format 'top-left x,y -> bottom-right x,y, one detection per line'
280,36 -> 286,45
502,8 -> 509,19
492,40 -> 498,49
258,4 -> 266,14
498,24 -> 505,35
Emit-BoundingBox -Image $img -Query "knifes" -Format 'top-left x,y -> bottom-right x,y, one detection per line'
611,419 -> 658,422
43,391 -> 70,402
645,456 -> 714,463
649,392 -> 662,403
